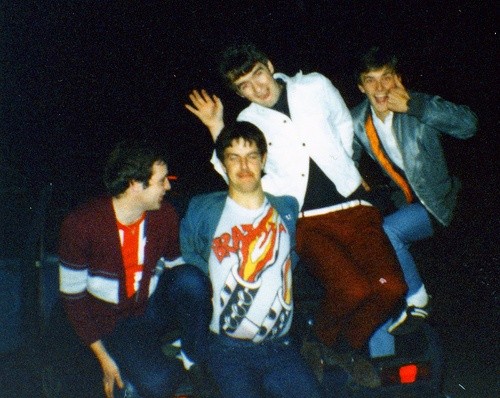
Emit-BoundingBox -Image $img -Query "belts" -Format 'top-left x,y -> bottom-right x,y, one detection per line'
298,199 -> 374,219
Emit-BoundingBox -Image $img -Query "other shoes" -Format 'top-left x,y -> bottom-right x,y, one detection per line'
300,329 -> 324,386
177,349 -> 220,398
323,344 -> 382,389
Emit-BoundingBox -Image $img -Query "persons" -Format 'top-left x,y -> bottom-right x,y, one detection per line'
346,46 -> 479,334
58,137 -> 213,398
179,121 -> 315,398
185,43 -> 366,223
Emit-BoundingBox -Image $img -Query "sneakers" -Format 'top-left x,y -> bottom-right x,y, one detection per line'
388,296 -> 435,336
